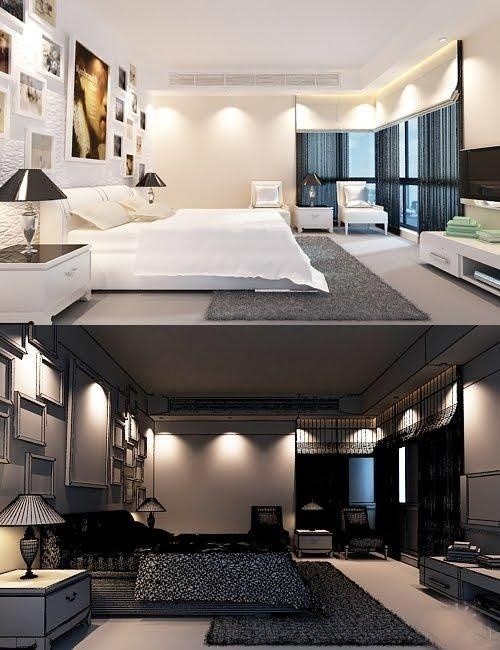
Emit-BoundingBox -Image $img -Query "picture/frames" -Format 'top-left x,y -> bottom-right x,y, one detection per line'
28,1 -> 61,36
134,458 -> 144,483
138,110 -> 146,132
0,348 -> 13,407
135,135 -> 143,159
128,414 -> 139,442
114,387 -> 128,420
113,96 -> 126,126
123,477 -> 134,504
0,323 -> 30,354
24,128 -> 56,171
124,153 -> 135,177
37,31 -> 65,84
0,86 -> 11,138
137,435 -> 147,457
129,63 -> 138,91
64,34 -> 113,165
12,65 -> 48,121
35,352 -> 63,409
12,390 -> 47,447
0,22 -> 12,81
111,456 -> 124,486
0,0 -> 28,34
22,452 -> 57,501
111,417 -> 127,450
124,115 -> 135,142
29,325 -> 60,360
64,358 -> 111,492
124,440 -> 135,468
115,63 -> 129,97
136,486 -> 146,509
0,410 -> 11,465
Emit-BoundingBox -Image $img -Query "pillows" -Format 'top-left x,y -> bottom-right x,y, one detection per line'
346,201 -> 372,208
344,185 -> 367,203
119,531 -> 149,551
132,203 -> 171,218
69,200 -> 132,231
119,198 -> 146,213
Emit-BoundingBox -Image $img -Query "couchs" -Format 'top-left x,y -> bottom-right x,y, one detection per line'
249,504 -> 290,550
339,504 -> 387,561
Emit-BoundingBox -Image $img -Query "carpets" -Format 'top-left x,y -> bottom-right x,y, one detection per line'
204,560 -> 431,646
204,236 -> 429,322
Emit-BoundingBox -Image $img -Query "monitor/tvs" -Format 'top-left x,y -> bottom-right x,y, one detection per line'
465,471 -> 499,528
456,145 -> 500,213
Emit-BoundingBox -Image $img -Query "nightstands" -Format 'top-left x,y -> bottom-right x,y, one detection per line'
0,245 -> 91,324
292,204 -> 334,234
294,529 -> 333,558
1,567 -> 94,649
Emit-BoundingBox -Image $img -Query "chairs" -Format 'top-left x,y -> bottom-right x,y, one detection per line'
248,179 -> 291,228
336,181 -> 389,236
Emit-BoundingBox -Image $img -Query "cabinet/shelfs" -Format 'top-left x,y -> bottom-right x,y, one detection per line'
419,230 -> 500,297
419,555 -> 500,621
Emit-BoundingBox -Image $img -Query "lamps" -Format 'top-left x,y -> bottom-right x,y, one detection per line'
0,494 -> 66,580
0,169 -> 67,255
303,174 -> 321,207
302,500 -> 323,531
134,498 -> 166,529
135,173 -> 167,206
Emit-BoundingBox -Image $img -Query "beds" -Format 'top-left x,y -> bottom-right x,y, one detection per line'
38,186 -> 313,291
36,507 -> 312,617
